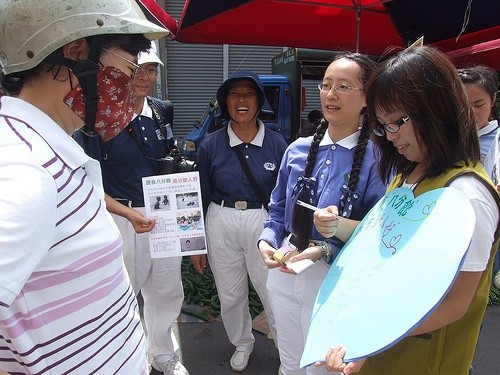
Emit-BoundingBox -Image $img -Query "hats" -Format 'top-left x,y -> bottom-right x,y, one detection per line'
216,71 -> 274,119
137,40 -> 165,67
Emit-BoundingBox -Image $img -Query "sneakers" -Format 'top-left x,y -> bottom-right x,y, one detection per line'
163,361 -> 189,374
229,343 -> 254,371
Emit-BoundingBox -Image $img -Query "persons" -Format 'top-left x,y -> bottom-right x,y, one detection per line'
75,40 -> 190,375
458,66 -> 500,185
189,70 -> 289,372
0,0 -> 168,375
258,53 -> 398,375
310,46 -> 500,375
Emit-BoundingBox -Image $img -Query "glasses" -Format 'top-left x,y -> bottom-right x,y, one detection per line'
136,67 -> 159,77
100,47 -> 141,83
317,83 -> 366,95
373,113 -> 414,137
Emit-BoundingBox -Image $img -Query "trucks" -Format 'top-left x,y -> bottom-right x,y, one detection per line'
181,48 -> 352,164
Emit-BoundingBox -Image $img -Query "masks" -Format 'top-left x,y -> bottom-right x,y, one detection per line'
62,65 -> 137,142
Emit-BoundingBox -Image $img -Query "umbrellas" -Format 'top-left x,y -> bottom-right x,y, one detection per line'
176,0 -> 500,57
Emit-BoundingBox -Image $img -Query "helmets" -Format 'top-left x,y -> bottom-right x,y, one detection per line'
0,1 -> 170,77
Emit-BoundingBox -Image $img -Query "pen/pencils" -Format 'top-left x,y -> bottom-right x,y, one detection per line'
297,199 -> 343,222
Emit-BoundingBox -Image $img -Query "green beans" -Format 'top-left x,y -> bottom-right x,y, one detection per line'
180,256 -> 265,322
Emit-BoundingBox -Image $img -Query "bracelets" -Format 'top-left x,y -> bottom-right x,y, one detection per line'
320,246 -> 326,261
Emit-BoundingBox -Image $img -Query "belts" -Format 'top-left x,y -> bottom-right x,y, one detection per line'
284,232 -> 316,252
211,197 -> 263,210
117,199 -> 146,208
